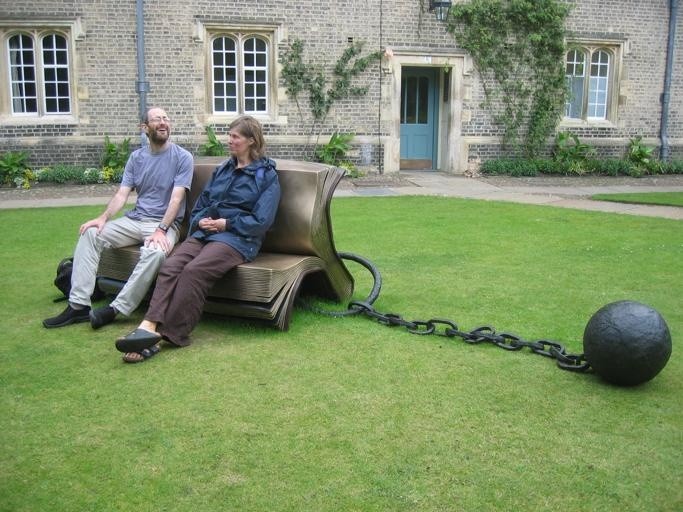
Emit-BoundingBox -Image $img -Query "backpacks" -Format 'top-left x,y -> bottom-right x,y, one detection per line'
52,258 -> 103,303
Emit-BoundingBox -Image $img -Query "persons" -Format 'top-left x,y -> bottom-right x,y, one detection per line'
42,108 -> 193,328
116,115 -> 281,364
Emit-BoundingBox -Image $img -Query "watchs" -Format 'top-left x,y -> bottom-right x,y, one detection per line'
158,224 -> 169,233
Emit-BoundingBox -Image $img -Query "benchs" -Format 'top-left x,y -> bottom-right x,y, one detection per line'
94,156 -> 356,332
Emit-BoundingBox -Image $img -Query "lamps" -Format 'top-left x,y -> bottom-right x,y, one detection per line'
428,0 -> 452,21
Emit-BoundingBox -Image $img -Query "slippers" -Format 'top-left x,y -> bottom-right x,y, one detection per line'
115,328 -> 162,364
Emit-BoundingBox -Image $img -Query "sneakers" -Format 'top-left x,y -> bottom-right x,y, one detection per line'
88,306 -> 115,328
43,305 -> 89,329
